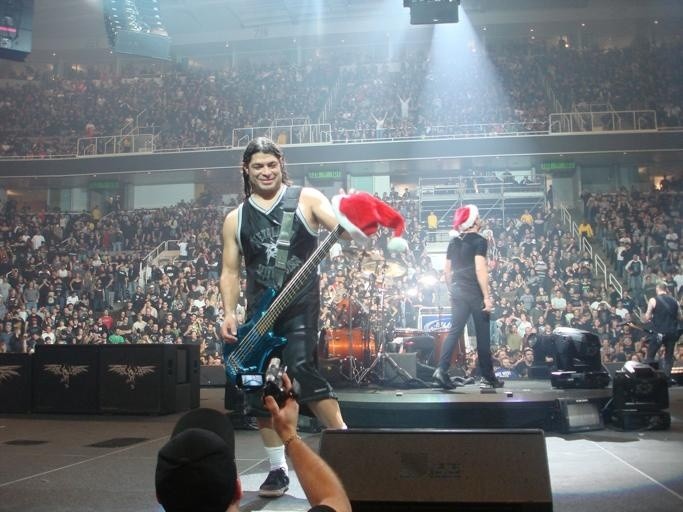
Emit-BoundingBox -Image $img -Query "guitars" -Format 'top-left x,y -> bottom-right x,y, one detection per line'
626,322 -> 683,342
222,223 -> 344,393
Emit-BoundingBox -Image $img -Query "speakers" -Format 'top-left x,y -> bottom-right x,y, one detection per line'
319,428 -> 554,512
105,1 -> 171,61
98,343 -> 200,415
0,352 -> 32,414
31,344 -> 98,415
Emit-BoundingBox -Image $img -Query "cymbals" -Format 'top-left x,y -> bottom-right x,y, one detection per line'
362,260 -> 406,278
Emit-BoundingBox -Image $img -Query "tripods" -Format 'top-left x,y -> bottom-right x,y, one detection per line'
356,273 -> 413,388
332,293 -> 370,386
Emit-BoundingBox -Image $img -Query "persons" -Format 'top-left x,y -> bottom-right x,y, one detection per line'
639,280 -> 682,387
1,39 -> 683,379
215,133 -> 362,501
149,368 -> 353,510
426,202 -> 506,391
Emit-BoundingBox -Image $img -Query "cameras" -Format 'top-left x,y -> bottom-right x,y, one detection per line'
236,351 -> 290,409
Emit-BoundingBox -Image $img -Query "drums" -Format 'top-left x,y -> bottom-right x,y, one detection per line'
332,293 -> 369,328
427,327 -> 467,369
393,327 -> 431,341
319,325 -> 377,384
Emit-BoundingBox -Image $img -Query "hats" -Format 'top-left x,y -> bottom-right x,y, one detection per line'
330,190 -> 409,253
449,203 -> 479,238
155,407 -> 237,512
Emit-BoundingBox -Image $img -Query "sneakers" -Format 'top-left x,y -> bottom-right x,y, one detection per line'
432,368 -> 456,390
258,466 -> 290,497
480,378 -> 505,388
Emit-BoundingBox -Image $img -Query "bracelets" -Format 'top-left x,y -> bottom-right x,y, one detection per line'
281,434 -> 304,460
